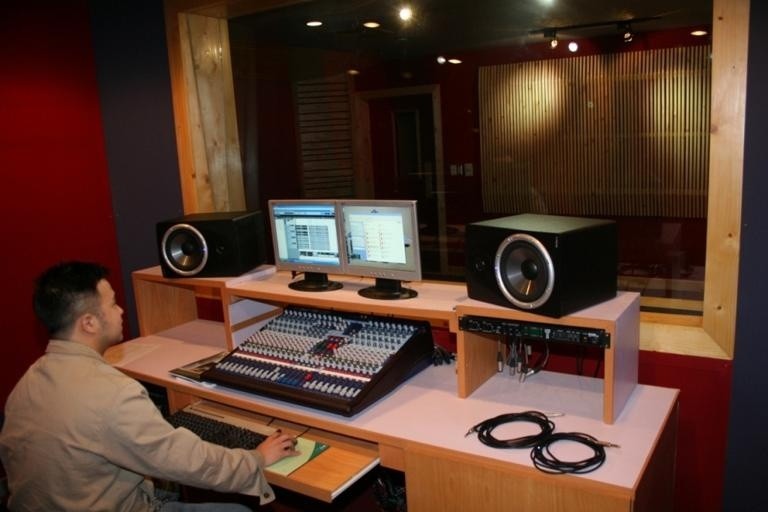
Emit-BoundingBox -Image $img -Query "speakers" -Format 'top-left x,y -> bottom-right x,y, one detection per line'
464,210 -> 618,319
153,208 -> 270,278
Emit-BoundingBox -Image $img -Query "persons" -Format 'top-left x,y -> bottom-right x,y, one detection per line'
0,257 -> 304,512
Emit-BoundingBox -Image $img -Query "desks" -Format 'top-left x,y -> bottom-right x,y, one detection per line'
103,318 -> 682,512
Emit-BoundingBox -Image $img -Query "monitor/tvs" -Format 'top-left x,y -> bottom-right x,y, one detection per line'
339,196 -> 425,300
267,196 -> 345,293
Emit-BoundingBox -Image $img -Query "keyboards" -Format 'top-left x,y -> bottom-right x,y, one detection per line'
165,407 -> 269,449
